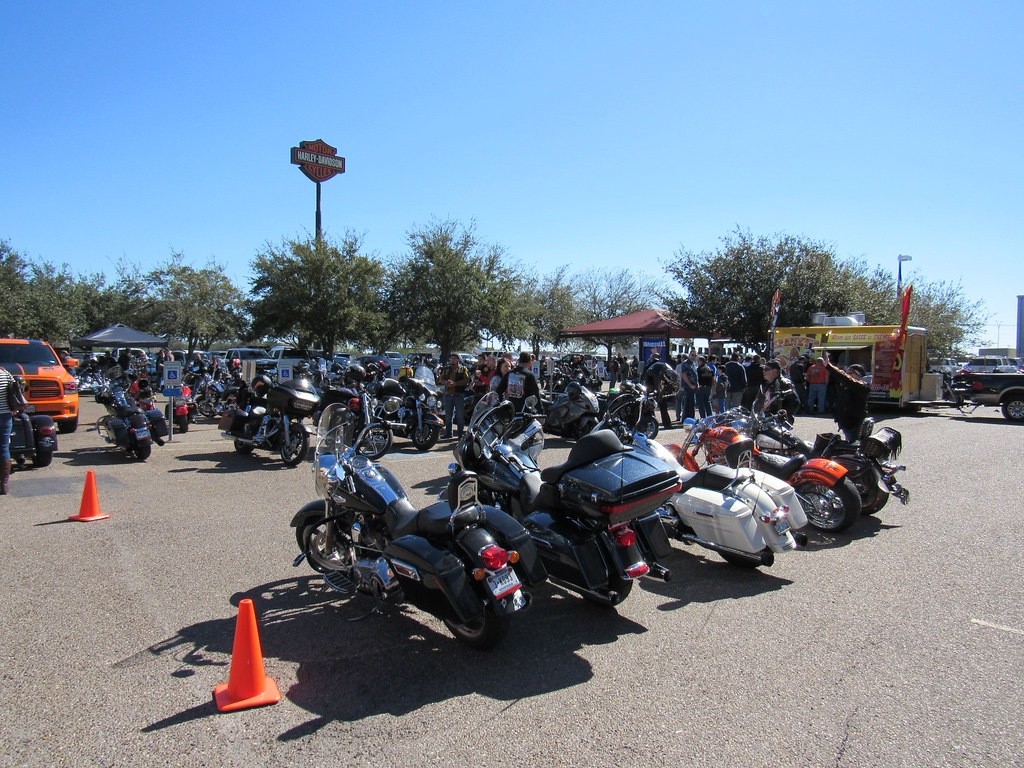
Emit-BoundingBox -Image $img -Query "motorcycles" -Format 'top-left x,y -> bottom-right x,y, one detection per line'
588,382 -> 807,571
8,375 -> 58,468
290,403 -> 555,654
746,383 -> 910,515
435,390 -> 682,610
72,355 -> 910,469
660,406 -> 862,535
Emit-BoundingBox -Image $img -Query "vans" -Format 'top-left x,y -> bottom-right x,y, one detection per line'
480,351 -> 521,367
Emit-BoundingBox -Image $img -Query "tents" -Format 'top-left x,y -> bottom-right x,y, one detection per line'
69,322 -> 168,383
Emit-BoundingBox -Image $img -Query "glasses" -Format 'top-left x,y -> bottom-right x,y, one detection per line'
761,367 -> 773,371
691,353 -> 697,357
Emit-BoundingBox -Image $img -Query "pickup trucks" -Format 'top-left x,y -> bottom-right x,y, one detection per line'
965,372 -> 1024,423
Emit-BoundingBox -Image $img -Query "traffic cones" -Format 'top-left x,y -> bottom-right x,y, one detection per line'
212,598 -> 282,712
67,471 -> 110,523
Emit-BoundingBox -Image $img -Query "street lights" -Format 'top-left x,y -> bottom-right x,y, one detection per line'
897,253 -> 913,298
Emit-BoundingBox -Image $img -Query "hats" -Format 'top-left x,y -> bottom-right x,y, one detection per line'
848,364 -> 866,377
767,360 -> 782,369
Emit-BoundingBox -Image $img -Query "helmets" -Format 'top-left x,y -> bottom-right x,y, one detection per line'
620,380 -> 632,389
347,364 -> 367,381
251,375 -> 272,398
14,376 -> 25,385
233,359 -> 241,367
645,363 -> 680,398
565,382 -> 582,401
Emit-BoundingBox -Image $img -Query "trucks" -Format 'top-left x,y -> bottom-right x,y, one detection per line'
772,316 -> 957,416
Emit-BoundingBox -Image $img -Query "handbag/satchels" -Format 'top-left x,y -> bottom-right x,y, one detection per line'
0,368 -> 27,410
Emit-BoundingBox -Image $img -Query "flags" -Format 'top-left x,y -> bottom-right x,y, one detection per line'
890,285 -> 913,398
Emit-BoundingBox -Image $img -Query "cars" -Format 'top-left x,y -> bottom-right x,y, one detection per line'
554,352 -> 584,367
171,349 -> 226,366
307,349 -> 333,362
451,353 -> 479,365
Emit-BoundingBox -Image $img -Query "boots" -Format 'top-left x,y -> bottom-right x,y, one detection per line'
0,459 -> 12,495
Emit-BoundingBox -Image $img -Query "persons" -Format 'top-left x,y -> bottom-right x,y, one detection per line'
61,351 -> 76,375
207,355 -> 222,380
230,358 -> 242,385
822,352 -> 870,442
82,346 -> 134,378
467,354 -> 488,406
154,348 -> 174,388
439,353 -> 471,439
489,358 -> 511,401
791,354 -> 838,413
724,353 -> 801,416
476,355 -> 497,383
530,354 -> 550,389
187,352 -> 210,404
497,352 -> 544,416
0,366 -> 17,495
605,353 -> 639,389
641,350 -> 731,430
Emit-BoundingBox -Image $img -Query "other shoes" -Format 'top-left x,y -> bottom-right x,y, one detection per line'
441,434 -> 453,439
458,436 -> 462,440
664,424 -> 671,428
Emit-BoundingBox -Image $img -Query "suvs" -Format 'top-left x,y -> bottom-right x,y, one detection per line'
927,355 -> 1024,374
225,348 -> 278,373
334,352 -> 351,367
112,348 -> 149,362
0,338 -> 80,433
267,345 -> 310,369
386,351 -> 405,365
